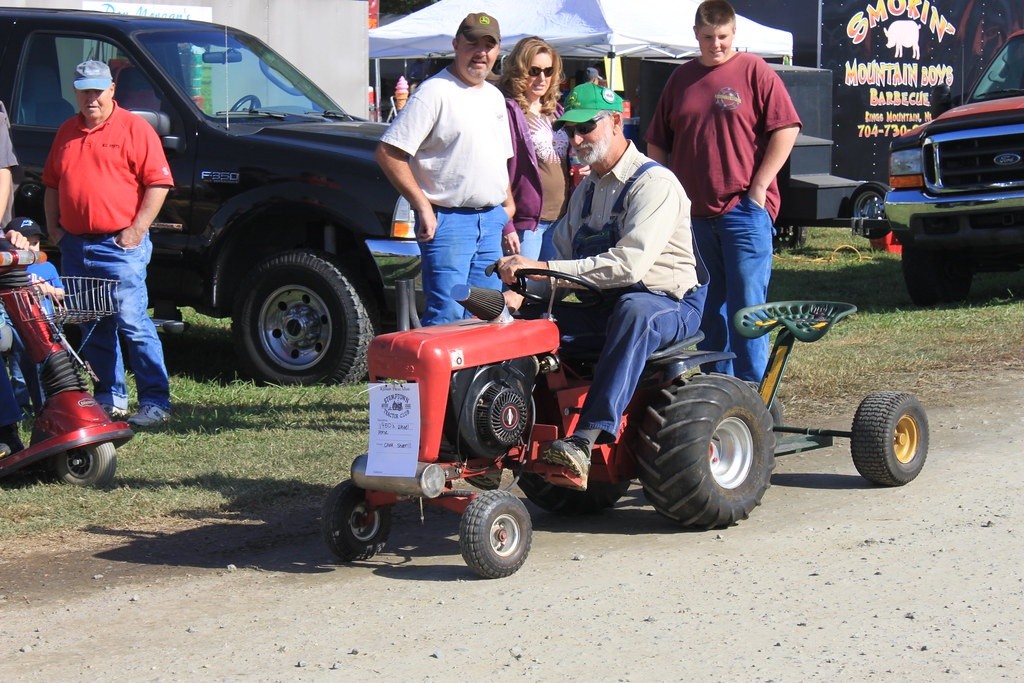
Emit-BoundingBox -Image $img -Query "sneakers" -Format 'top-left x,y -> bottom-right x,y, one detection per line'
101,403 -> 126,419
545,438 -> 592,492
128,405 -> 170,425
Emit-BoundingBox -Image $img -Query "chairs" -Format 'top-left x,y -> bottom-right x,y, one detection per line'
21,64 -> 76,127
113,65 -> 160,111
559,330 -> 706,361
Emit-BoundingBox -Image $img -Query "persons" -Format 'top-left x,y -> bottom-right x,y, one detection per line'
0,111 -> 31,253
374,12 -> 515,328
5,217 -> 66,414
643,0 -> 803,382
583,68 -> 609,85
498,82 -> 710,492
0,230 -> 30,444
42,58 -> 174,425
494,36 -> 572,314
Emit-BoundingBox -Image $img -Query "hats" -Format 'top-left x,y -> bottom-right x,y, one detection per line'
551,82 -> 623,132
73,60 -> 112,90
5,217 -> 46,238
458,12 -> 502,45
579,67 -> 602,80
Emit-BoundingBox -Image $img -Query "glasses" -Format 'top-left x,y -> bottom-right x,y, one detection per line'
528,65 -> 556,78
563,113 -> 610,139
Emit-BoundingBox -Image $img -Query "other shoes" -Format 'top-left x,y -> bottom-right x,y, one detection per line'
0,437 -> 24,459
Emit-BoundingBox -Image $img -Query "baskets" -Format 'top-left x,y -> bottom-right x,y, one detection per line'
12,277 -> 121,322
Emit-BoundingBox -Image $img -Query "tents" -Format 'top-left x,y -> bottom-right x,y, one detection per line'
369,0 -> 793,119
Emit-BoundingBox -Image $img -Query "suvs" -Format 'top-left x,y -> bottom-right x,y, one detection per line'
0,7 -> 421,387
883,29 -> 1024,309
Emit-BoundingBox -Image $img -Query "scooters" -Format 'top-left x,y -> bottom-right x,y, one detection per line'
0,238 -> 132,490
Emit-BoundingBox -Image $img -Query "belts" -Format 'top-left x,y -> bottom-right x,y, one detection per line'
80,232 -> 118,240
